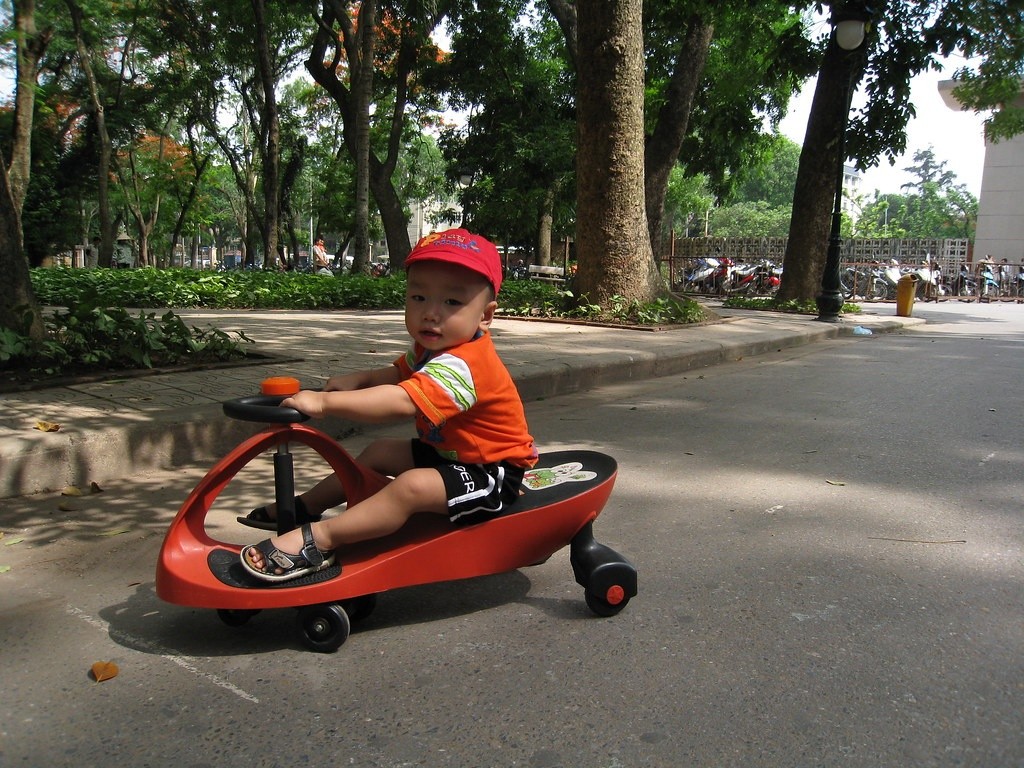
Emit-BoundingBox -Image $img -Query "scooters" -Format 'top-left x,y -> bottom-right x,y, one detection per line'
675,254 -> 1024,304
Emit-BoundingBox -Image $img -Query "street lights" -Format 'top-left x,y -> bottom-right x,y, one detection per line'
369,243 -> 373,262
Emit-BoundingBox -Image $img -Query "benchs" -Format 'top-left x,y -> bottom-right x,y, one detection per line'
528,264 -> 566,289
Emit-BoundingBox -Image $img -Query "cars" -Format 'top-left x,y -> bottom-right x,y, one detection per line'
199,247 -> 209,255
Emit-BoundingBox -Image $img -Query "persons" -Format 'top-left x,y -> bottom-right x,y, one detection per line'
313,237 -> 331,275
240,229 -> 539,581
1019,258 -> 1024,274
1001,258 -> 1010,274
989,255 -> 995,270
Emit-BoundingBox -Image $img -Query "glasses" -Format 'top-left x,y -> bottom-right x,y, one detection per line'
319,240 -> 324,243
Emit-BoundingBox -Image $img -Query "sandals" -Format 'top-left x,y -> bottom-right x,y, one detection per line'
237,495 -> 322,531
239,522 -> 336,582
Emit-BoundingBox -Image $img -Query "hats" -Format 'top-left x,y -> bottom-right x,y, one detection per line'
403,228 -> 503,300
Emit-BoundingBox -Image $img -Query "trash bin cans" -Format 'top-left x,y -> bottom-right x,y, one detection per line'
896,274 -> 919,317
